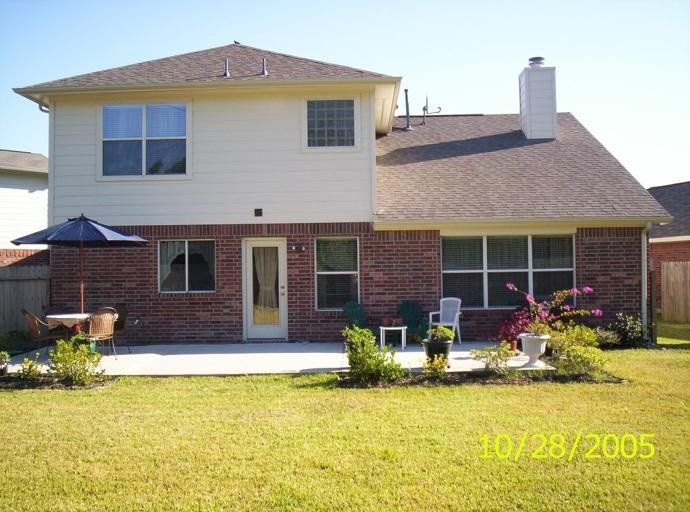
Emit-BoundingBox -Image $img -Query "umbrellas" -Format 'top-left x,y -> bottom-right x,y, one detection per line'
10,211 -> 150,313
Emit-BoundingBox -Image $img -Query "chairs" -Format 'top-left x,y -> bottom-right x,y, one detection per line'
343,297 -> 463,346
20,302 -> 132,360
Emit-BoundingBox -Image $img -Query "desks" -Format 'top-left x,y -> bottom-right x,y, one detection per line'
378,325 -> 407,350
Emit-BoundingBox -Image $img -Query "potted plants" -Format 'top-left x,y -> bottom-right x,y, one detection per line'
421,325 -> 456,360
517,318 -> 551,369
